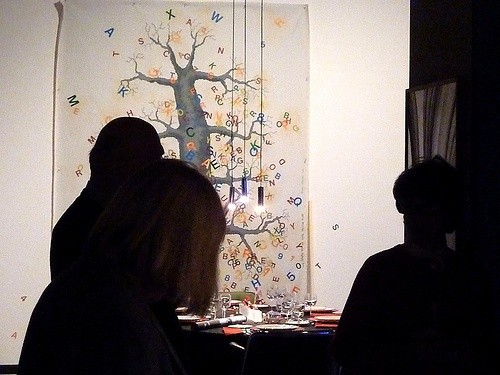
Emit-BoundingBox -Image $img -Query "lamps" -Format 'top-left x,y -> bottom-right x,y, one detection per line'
256,0 -> 264,215
227,0 -> 237,212
241,0 -> 248,204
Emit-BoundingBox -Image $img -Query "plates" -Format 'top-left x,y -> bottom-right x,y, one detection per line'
315,316 -> 341,321
285,321 -> 310,324
228,324 -> 253,329
256,324 -> 299,329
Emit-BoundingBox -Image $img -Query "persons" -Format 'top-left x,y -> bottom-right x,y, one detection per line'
330,159 -> 500,375
17,116 -> 225,375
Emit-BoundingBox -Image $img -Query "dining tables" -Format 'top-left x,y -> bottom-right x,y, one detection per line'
181,314 -> 337,375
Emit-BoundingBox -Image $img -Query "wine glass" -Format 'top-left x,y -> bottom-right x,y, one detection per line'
266,289 -> 317,328
211,293 -> 232,319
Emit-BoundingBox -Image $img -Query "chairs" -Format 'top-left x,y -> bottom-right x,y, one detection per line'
229,331 -> 345,375
215,292 -> 255,307
